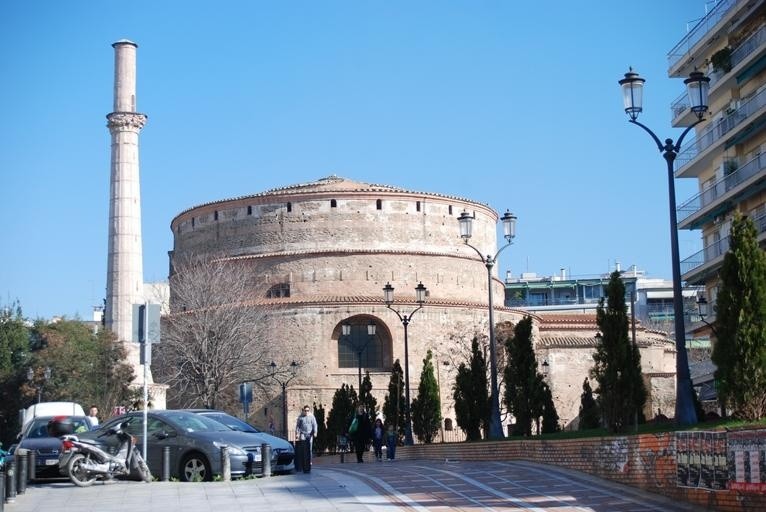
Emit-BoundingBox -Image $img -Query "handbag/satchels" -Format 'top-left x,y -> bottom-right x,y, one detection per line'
348,411 -> 358,435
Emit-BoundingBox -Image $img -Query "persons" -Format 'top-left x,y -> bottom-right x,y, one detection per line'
295,405 -> 318,465
384,424 -> 399,460
349,402 -> 371,463
86,405 -> 98,426
371,419 -> 384,462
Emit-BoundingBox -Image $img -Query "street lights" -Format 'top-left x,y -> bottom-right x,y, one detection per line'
24,364 -> 51,402
617,61 -> 713,430
382,280 -> 426,447
456,205 -> 516,444
340,318 -> 378,398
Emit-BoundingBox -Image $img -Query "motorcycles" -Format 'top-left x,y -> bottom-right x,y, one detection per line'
56,400 -> 153,487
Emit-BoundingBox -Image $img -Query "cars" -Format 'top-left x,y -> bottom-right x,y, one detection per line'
74,409 -> 279,483
185,409 -> 296,474
3,444 -> 19,470
15,417 -> 95,482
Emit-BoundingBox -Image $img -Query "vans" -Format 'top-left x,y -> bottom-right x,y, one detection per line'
20,400 -> 86,434
